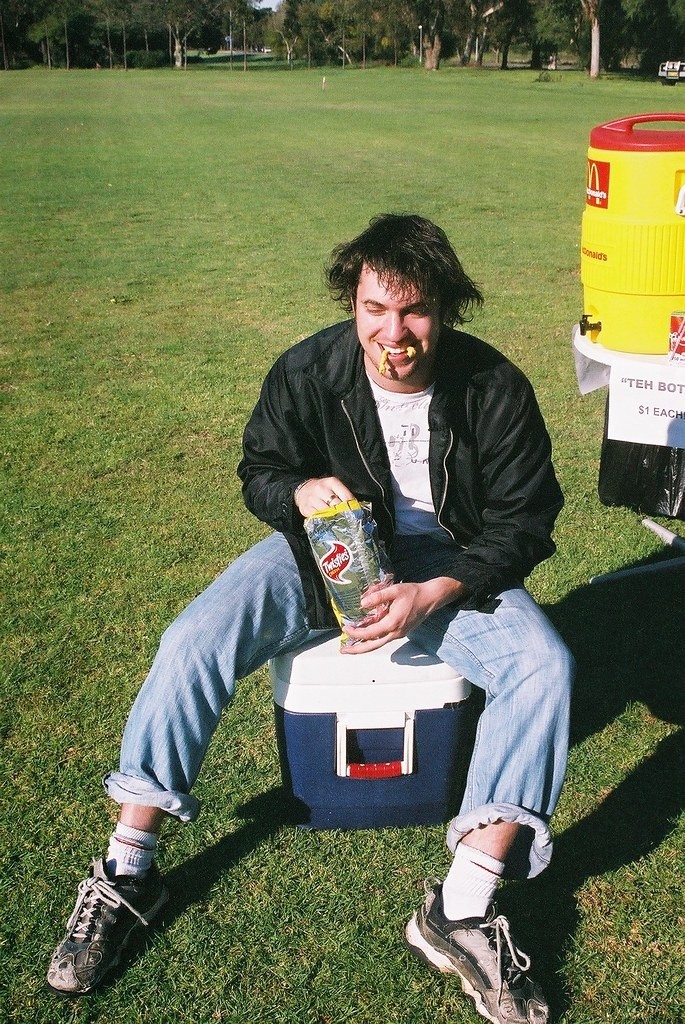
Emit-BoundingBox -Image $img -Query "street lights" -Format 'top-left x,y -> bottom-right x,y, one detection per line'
418,25 -> 424,65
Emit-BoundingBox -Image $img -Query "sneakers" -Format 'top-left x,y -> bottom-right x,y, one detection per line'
404,876 -> 549,1024
46,854 -> 167,991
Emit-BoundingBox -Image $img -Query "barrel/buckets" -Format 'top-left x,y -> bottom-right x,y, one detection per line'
578,113 -> 685,354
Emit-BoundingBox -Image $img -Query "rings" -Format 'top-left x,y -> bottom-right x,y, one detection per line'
326,495 -> 337,505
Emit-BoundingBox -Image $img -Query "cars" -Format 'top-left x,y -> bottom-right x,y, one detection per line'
658,54 -> 685,86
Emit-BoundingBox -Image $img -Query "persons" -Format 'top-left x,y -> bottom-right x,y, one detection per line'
46,212 -> 578,1024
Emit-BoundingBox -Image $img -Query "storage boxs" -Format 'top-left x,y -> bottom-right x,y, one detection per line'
268,628 -> 473,831
